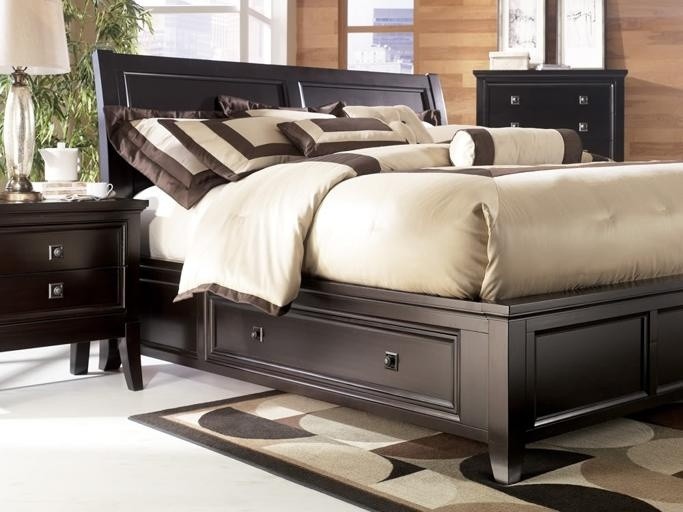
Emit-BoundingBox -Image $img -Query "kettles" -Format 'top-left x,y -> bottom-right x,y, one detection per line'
38,141 -> 81,182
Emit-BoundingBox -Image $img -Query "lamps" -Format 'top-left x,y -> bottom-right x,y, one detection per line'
2,2 -> 72,202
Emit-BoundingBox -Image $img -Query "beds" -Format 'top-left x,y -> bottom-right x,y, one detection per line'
90,48 -> 683,486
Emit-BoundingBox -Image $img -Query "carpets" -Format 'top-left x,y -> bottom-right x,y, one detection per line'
127,385 -> 682,511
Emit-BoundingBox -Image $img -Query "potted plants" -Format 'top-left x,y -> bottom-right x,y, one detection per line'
1,1 -> 158,183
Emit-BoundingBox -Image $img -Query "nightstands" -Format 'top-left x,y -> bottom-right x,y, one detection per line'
470,69 -> 627,166
1,183 -> 149,391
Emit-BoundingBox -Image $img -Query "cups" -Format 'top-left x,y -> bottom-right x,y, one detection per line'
86,181 -> 113,198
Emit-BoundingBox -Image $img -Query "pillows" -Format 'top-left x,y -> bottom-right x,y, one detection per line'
280,116 -> 409,157
228,109 -> 337,125
102,106 -> 229,210
341,105 -> 434,143
449,126 -> 583,167
157,114 -> 305,183
